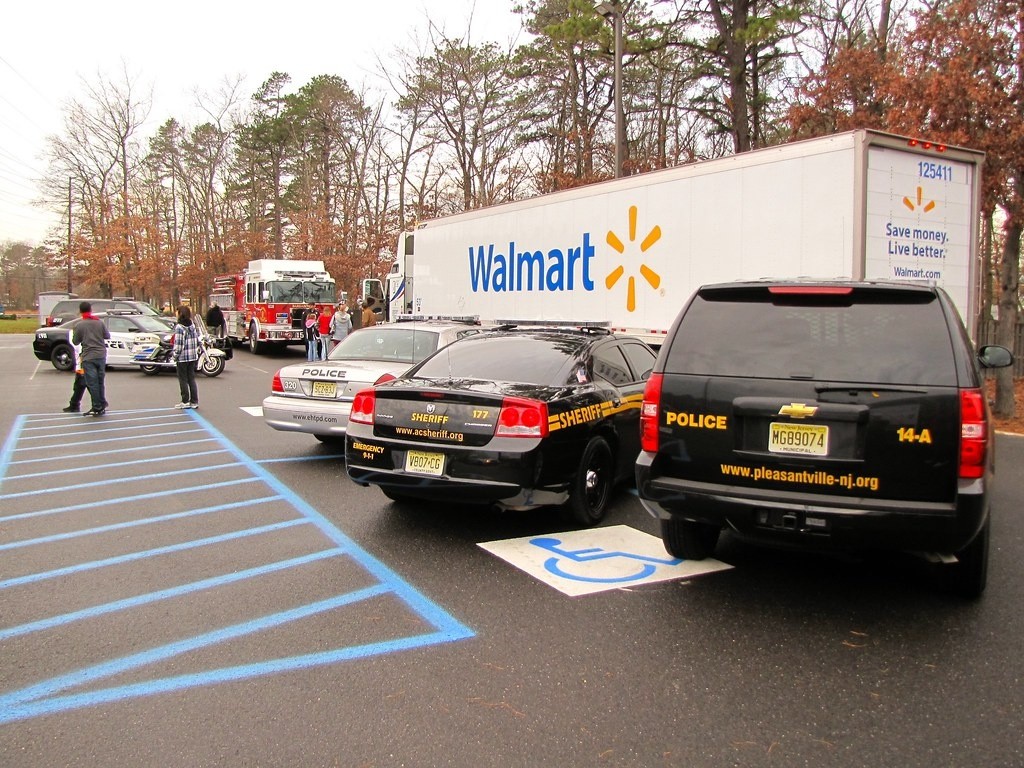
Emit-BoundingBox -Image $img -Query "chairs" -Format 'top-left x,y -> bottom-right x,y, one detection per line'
766,316 -> 810,340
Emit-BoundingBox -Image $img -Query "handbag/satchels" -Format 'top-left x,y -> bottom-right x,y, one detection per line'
312,327 -> 321,342
328,314 -> 336,335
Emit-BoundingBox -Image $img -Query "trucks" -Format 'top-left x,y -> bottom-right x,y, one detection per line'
39,292 -> 80,327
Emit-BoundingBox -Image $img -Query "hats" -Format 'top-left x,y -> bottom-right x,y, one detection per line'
307,301 -> 315,305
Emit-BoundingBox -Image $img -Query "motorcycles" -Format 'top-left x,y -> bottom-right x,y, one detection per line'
129,313 -> 227,377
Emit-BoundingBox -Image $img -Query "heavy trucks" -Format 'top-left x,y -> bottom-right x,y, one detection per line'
361,129 -> 984,354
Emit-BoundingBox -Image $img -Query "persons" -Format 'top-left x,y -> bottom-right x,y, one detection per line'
171,306 -> 199,410
206,301 -> 224,335
301,299 -> 376,361
63,302 -> 111,417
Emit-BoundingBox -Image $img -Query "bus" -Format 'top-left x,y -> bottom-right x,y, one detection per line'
181,299 -> 195,306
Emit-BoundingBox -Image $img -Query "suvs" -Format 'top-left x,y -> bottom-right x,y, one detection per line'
45,299 -> 177,332
634,275 -> 1013,601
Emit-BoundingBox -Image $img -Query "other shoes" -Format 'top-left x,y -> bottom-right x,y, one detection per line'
83,408 -> 105,417
190,403 -> 199,409
63,406 -> 80,412
104,401 -> 108,407
174,402 -> 191,409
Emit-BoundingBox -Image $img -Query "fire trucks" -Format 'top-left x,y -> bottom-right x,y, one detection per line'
208,258 -> 339,354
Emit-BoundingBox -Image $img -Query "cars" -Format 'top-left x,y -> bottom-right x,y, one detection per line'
261,319 -> 497,444
33,311 -> 233,371
344,330 -> 660,524
164,302 -> 172,307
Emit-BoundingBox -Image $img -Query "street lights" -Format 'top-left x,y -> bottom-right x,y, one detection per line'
594,1 -> 623,179
68,177 -> 76,293
